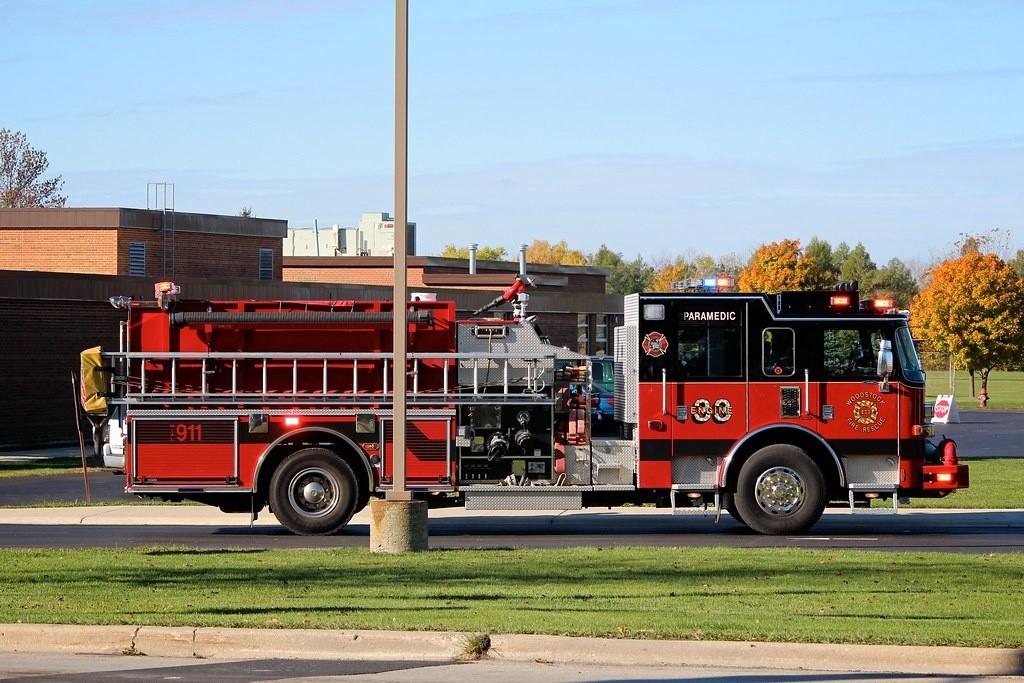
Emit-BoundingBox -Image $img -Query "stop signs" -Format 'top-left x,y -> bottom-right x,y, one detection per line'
932,400 -> 950,419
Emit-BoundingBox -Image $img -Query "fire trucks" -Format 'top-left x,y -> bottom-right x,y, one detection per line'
68,243 -> 972,539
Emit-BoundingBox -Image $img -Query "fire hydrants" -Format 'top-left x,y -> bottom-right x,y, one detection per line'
977,388 -> 991,408
